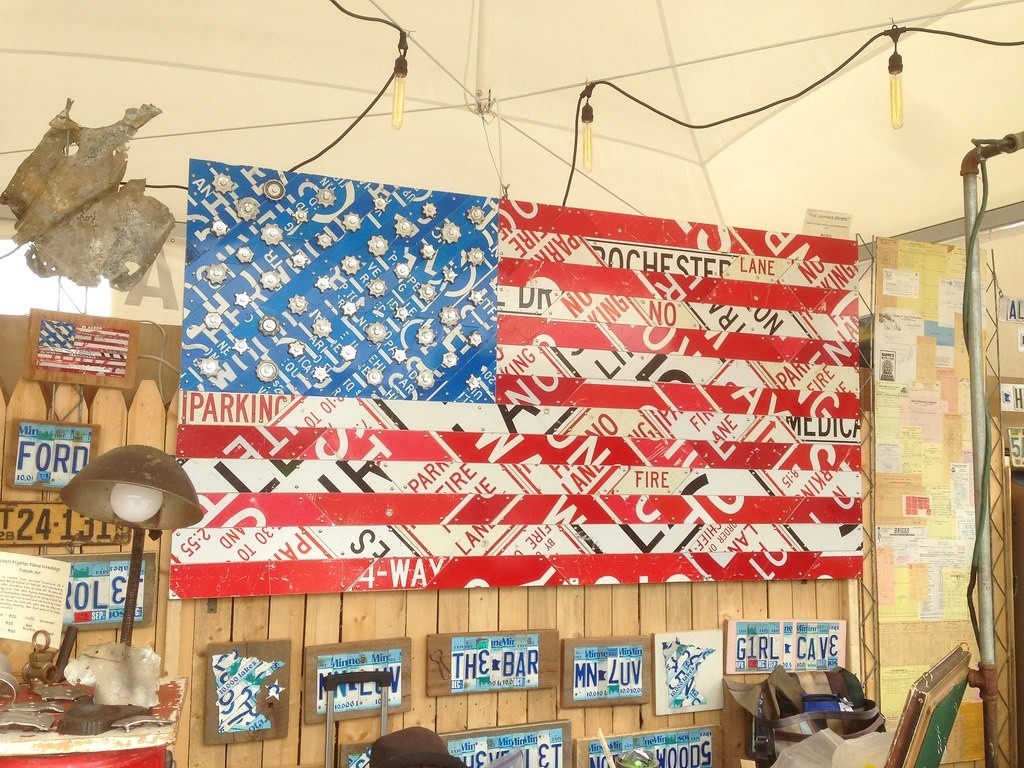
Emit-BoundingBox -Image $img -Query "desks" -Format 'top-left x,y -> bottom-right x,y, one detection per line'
0,675 -> 187,768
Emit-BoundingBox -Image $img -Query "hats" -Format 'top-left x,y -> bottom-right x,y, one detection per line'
370,727 -> 468,768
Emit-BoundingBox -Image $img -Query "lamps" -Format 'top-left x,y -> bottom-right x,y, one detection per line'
60,445 -> 205,736
